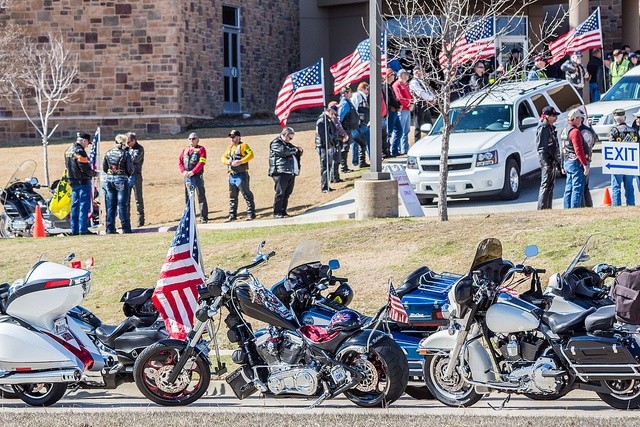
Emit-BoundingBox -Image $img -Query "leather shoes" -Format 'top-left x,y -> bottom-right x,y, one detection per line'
80,230 -> 97,235
272,213 -> 294,219
243,214 -> 256,221
137,221 -> 144,227
223,216 -> 237,223
321,187 -> 335,194
200,218 -> 209,224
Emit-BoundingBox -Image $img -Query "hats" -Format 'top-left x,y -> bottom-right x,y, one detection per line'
228,129 -> 243,136
542,106 -> 561,116
612,49 -> 625,57
77,132 -> 93,145
396,69 -> 412,76
535,56 -> 548,62
567,108 -> 586,118
329,101 -> 341,108
611,109 -> 627,117
629,52 -> 640,61
572,51 -> 586,57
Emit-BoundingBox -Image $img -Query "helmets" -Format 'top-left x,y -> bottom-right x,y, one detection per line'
326,309 -> 363,334
321,283 -> 353,310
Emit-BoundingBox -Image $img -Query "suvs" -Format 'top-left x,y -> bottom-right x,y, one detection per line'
405,80 -> 585,205
578,66 -> 640,151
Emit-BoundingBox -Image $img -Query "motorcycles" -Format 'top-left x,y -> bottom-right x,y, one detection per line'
543,233 -> 640,311
250,240 -> 467,401
0,160 -> 102,242
417,238 -> 640,411
133,251 -> 409,409
0,283 -> 210,400
0,260 -> 91,408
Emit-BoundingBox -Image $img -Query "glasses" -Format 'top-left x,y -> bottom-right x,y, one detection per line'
478,66 -> 485,69
187,137 -> 197,140
286,135 -> 293,141
127,140 -> 133,144
364,86 -> 371,91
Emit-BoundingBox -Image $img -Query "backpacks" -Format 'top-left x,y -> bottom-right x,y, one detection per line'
608,265 -> 640,325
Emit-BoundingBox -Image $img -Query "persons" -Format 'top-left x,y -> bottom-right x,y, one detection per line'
527,56 -> 548,81
367,76 -> 390,159
381,68 -> 404,158
64,132 -> 99,235
350,81 -> 370,166
409,66 -> 437,142
560,107 -> 589,209
588,45 -> 640,98
560,51 -> 592,99
103,133 -> 135,234
536,106 -> 561,210
633,111 -> 640,193
609,109 -> 640,207
392,68 -> 411,154
469,62 -> 504,92
578,108 -> 598,207
267,127 -> 303,219
314,105 -> 343,193
337,86 -> 370,173
221,129 -> 256,223
178,132 -> 208,224
505,48 -> 526,83
118,131 -> 145,229
328,101 -> 349,183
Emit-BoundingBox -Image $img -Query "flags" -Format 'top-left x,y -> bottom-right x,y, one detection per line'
330,31 -> 387,96
497,285 -> 518,298
439,13 -> 495,81
66,130 -> 99,222
151,196 -> 206,342
48,169 -> 73,223
274,58 -> 324,129
543,8 -> 602,66
386,282 -> 413,328
